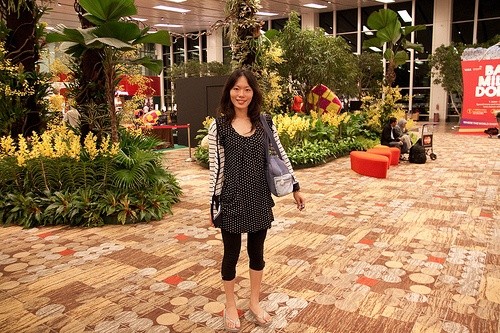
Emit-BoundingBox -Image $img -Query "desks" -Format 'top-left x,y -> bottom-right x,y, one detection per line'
139,125 -> 174,149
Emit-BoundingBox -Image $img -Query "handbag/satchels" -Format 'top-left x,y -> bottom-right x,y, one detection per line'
260,113 -> 300,198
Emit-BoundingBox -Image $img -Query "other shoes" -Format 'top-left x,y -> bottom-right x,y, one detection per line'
401,157 -> 408,161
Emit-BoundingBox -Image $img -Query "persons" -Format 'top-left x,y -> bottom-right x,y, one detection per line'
394,119 -> 414,152
381,117 -> 410,162
208,62 -> 307,330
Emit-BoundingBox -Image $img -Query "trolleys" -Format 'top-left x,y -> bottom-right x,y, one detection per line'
422,123 -> 437,160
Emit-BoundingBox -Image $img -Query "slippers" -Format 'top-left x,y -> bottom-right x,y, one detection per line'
248,305 -> 272,327
223,310 -> 241,332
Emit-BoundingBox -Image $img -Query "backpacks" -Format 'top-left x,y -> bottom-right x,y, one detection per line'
409,144 -> 427,164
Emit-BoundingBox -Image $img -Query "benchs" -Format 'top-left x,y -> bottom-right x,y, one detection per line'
349,144 -> 403,179
